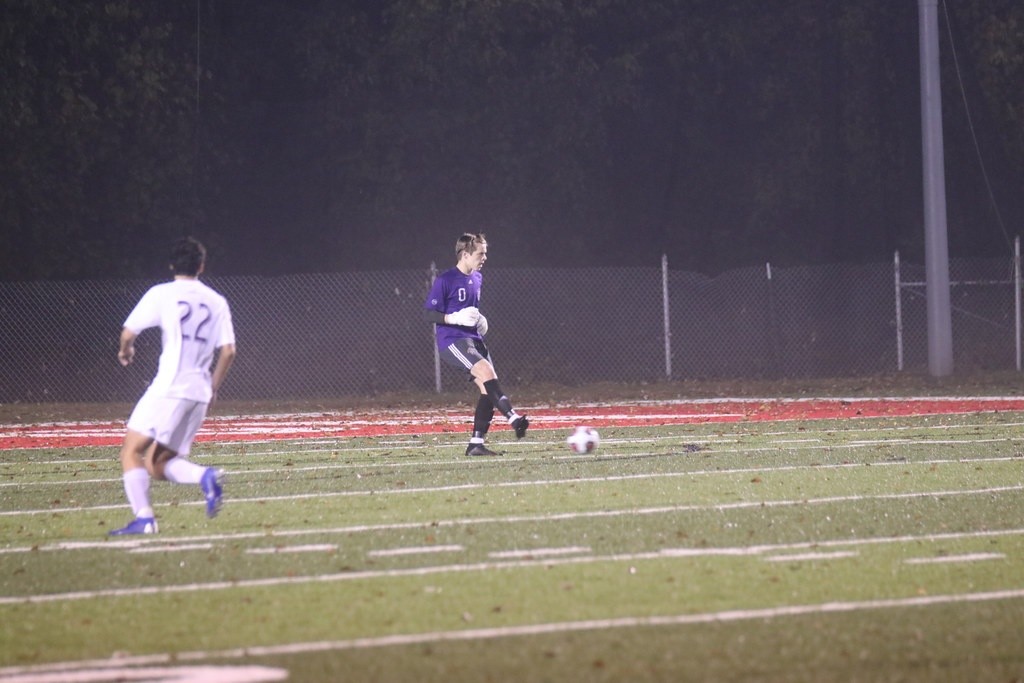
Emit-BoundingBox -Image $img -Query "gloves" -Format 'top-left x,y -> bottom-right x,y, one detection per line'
476,313 -> 488,336
446,306 -> 480,327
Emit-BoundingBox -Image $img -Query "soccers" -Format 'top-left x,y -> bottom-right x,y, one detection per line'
565,426 -> 600,456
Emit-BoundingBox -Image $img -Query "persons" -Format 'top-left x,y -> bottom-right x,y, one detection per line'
108,236 -> 236,535
421,232 -> 530,456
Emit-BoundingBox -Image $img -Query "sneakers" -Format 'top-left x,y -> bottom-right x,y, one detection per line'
108,517 -> 159,536
200,466 -> 225,519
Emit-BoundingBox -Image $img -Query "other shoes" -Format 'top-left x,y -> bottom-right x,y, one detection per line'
507,413 -> 529,439
465,438 -> 496,455
